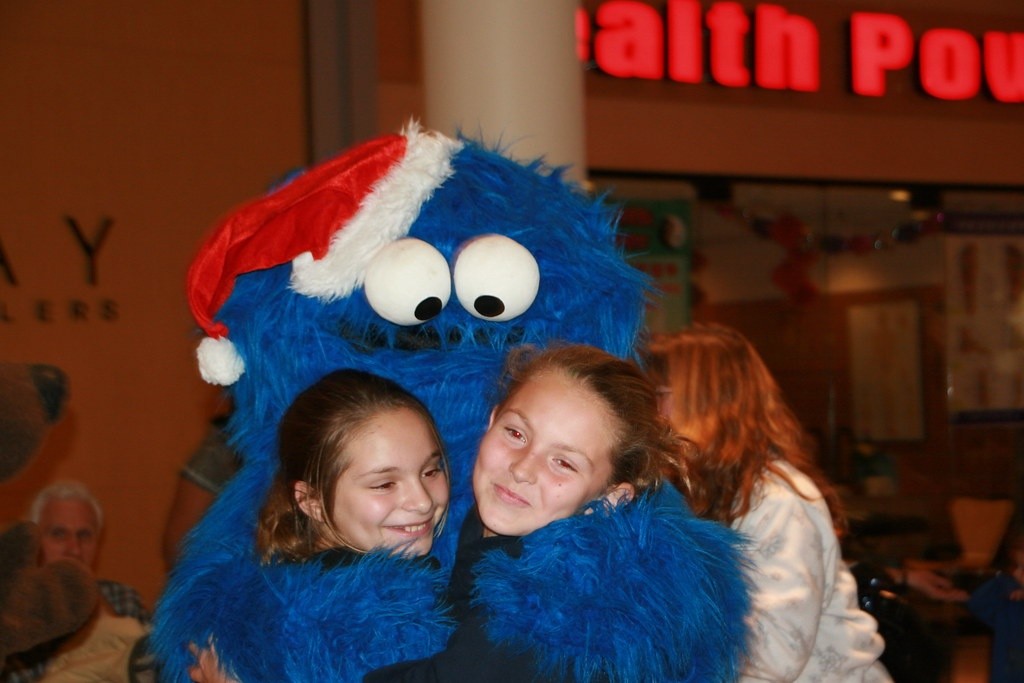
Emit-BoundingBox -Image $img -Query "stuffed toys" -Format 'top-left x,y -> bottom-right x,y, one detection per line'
0,354 -> 96,657
131,116 -> 752,683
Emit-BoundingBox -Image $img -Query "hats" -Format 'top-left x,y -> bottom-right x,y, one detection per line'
0,522 -> 98,670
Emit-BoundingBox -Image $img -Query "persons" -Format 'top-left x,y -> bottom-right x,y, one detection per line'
637,326 -> 897,683
188,343 -> 689,682
18,480 -> 156,683
802,425 -> 889,493
254,369 -> 454,576
967,534 -> 1023,683
